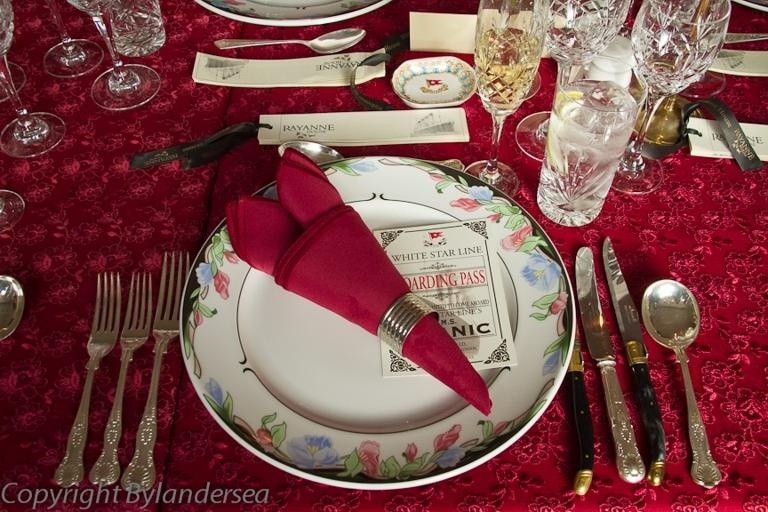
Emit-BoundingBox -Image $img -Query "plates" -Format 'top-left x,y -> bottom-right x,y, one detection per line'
389,55 -> 478,110
179,156 -> 576,491
191,0 -> 397,27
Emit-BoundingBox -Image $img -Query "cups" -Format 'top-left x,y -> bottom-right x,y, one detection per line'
537,80 -> 641,230
106,0 -> 166,57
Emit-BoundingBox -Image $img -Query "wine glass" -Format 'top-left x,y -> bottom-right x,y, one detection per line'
0,0 -> 161,157
463,0 -> 732,198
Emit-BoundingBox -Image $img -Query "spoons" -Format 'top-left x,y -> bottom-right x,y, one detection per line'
641,278 -> 722,490
213,27 -> 367,56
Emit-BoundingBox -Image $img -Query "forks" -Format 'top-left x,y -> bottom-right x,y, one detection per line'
54,250 -> 189,494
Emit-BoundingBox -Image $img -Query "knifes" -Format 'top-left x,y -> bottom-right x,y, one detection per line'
571,237 -> 667,497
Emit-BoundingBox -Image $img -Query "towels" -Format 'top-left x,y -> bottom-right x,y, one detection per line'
224,146 -> 493,416
